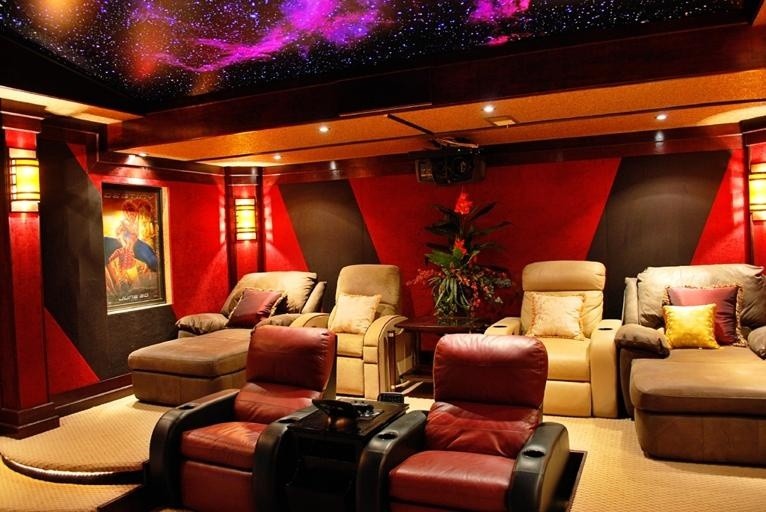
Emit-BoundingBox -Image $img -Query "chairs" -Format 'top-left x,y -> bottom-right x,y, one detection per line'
612,260 -> 765,468
122,268 -> 328,404
483,258 -> 621,423
351,334 -> 569,512
139,324 -> 339,511
289,262 -> 414,403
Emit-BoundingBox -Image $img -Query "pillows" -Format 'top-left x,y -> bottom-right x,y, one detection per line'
222,284 -> 289,326
331,292 -> 384,336
525,287 -> 588,343
660,302 -> 721,349
663,281 -> 751,351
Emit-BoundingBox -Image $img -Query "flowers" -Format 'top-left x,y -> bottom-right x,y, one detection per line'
413,186 -> 518,322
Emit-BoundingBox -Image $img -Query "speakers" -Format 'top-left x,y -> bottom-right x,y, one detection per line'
430,151 -> 485,186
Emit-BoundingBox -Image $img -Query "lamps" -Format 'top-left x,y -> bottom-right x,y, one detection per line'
746,161 -> 765,221
3,145 -> 41,214
233,196 -> 257,243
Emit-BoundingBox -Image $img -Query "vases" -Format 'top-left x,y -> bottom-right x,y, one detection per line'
433,305 -> 472,324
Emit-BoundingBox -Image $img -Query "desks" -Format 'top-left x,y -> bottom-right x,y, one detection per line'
286,392 -> 410,511
391,307 -> 498,393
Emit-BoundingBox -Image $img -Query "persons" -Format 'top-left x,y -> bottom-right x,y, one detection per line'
103,199 -> 158,281
